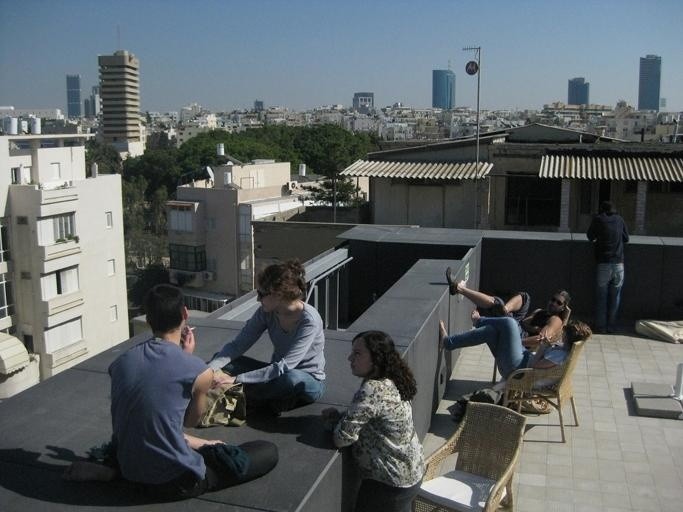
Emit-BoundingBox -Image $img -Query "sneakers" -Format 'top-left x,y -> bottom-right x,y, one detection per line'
446,267 -> 458,295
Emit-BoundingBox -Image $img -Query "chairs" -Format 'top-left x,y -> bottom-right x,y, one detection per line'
411,306 -> 587,512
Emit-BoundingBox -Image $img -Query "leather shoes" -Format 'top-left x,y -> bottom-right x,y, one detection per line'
596,325 -> 627,335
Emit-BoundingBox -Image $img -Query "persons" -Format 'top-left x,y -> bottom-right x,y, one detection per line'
584,200 -> 630,334
314,328 -> 428,512
106,283 -> 278,502
204,259 -> 326,420
438,307 -> 591,391
444,264 -> 572,350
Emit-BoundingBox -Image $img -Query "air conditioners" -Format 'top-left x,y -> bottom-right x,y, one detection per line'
203,271 -> 214,281
287,181 -> 297,191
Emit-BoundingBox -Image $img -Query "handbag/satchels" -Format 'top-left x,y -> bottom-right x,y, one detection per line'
200,382 -> 247,428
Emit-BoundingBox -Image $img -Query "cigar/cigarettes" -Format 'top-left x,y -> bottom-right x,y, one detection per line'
183,326 -> 196,333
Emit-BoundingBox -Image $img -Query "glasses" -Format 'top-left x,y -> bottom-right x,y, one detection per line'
257,289 -> 272,298
550,296 -> 563,306
